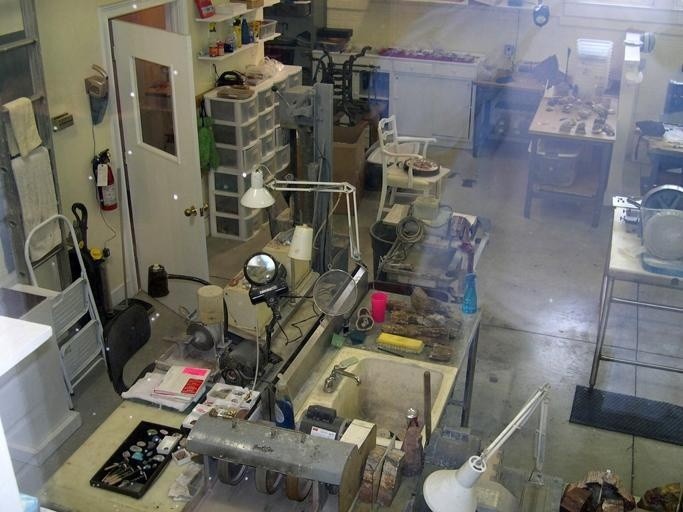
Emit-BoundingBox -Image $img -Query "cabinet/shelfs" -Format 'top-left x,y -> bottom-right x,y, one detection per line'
199,58 -> 306,243
194,0 -> 283,62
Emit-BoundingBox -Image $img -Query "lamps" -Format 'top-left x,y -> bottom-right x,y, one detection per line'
422,379 -> 551,512
531,3 -> 549,27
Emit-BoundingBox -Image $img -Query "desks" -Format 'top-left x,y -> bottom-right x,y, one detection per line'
469,63 -> 618,233
588,195 -> 683,394
36,262 -> 486,512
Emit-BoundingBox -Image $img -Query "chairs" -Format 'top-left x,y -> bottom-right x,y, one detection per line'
374,112 -> 449,225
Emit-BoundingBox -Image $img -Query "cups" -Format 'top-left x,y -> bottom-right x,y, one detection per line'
368,291 -> 388,324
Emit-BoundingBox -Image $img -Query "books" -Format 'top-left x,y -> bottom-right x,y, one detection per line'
151,365 -> 212,402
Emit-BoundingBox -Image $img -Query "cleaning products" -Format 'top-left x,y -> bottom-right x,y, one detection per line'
209,21 -> 219,56
460,273 -> 477,313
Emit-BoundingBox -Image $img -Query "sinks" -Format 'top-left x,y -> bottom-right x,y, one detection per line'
294,347 -> 458,451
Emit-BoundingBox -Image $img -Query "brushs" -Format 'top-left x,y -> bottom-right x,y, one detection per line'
375,331 -> 425,354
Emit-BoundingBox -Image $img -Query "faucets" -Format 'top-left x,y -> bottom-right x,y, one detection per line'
331,370 -> 361,386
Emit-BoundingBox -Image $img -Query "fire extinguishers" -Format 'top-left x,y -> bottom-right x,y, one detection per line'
93,148 -> 118,211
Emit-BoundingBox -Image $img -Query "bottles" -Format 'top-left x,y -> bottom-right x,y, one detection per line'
398,406 -> 424,478
206,22 -> 224,57
233,17 -> 250,49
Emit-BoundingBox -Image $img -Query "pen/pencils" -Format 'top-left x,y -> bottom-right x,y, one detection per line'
378,347 -> 404,358
